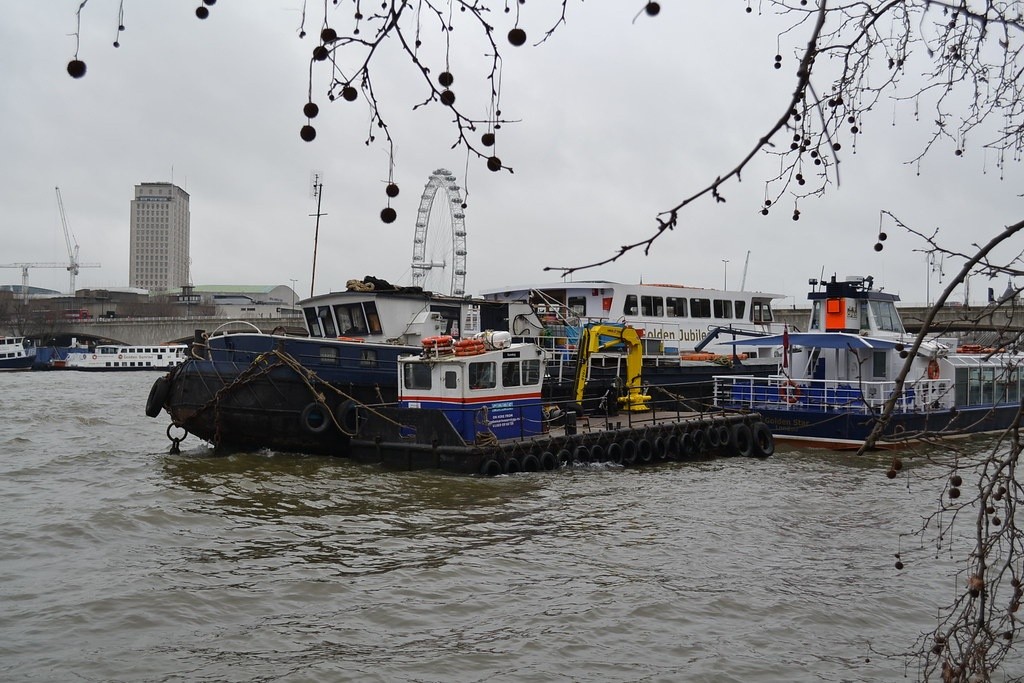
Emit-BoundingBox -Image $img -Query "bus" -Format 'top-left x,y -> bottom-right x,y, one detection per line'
30,308 -> 90,319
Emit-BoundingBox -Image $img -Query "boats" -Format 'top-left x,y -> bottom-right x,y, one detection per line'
0,335 -> 189,371
693,272 -> 1023,451
145,275 -> 804,455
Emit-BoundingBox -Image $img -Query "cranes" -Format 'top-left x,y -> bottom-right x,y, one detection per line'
1,262 -> 101,306
54,186 -> 80,297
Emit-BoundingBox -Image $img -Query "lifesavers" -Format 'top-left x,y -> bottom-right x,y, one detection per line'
928,360 -> 940,379
337,336 -> 365,341
118,354 -> 123,359
92,355 -> 97,359
453,336 -> 486,356
421,335 -> 453,347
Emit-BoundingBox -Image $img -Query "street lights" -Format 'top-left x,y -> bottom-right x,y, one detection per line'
721,259 -> 731,291
911,249 -> 936,310
289,278 -> 298,318
